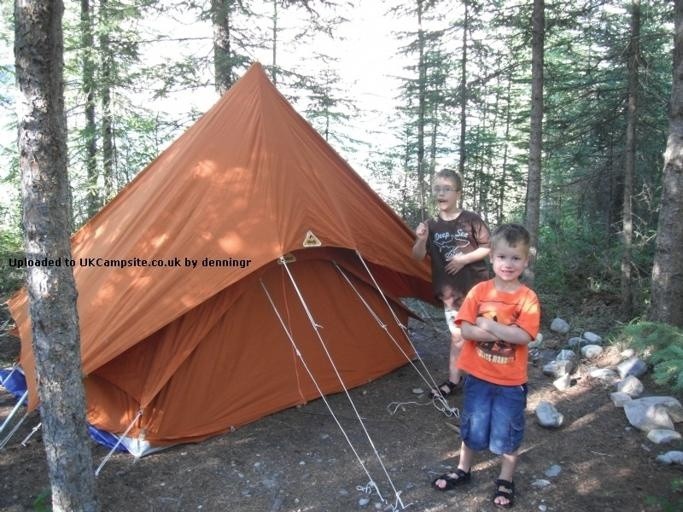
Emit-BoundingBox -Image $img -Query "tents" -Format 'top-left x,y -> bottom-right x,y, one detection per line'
5,60 -> 432,457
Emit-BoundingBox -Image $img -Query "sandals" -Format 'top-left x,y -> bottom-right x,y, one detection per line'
428,376 -> 464,400
430,465 -> 471,492
492,478 -> 514,509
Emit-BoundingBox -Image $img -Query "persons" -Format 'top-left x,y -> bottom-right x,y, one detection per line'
412,168 -> 494,401
431,223 -> 542,511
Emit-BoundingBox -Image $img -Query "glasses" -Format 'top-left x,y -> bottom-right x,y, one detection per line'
432,186 -> 462,195
